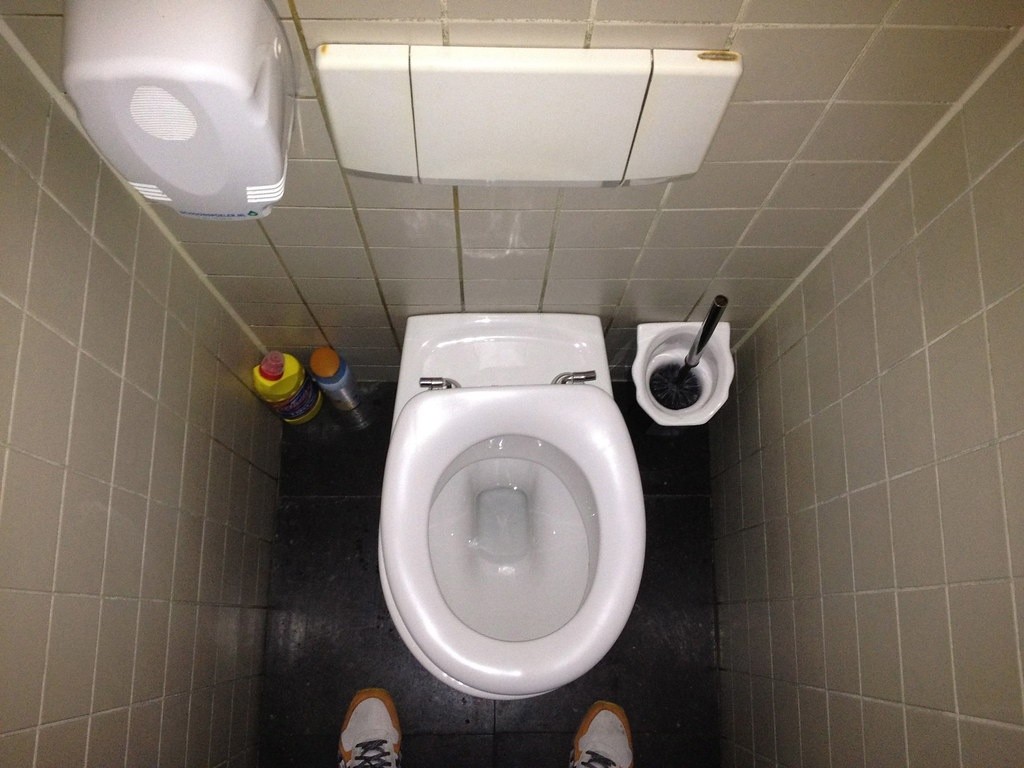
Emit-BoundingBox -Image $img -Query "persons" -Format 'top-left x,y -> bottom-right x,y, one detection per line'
329,686 -> 639,768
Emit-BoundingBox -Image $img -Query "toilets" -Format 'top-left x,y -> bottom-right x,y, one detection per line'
377,313 -> 648,698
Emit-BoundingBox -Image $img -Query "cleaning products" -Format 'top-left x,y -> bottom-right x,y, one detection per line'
312,349 -> 364,410
254,350 -> 322,423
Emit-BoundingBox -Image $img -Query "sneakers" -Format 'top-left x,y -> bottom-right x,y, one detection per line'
338,688 -> 403,768
569,702 -> 634,768
